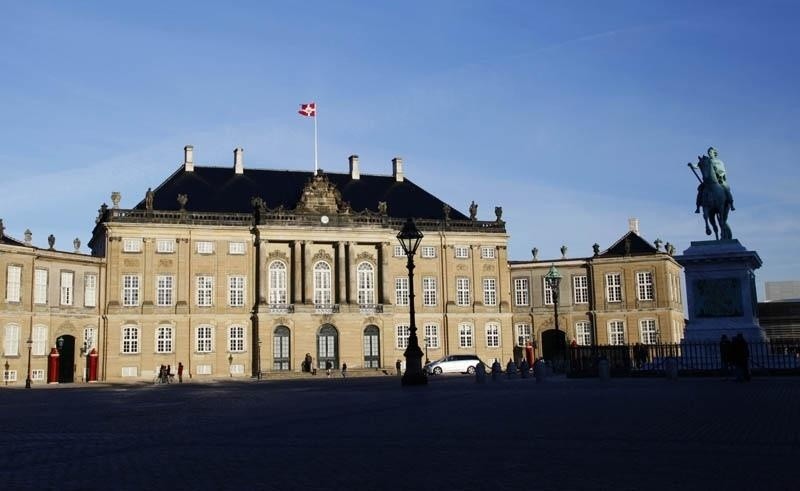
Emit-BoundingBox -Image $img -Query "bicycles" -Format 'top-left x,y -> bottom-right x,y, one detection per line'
154,369 -> 176,386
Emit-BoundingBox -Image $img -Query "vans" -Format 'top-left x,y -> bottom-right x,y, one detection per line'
422,353 -> 482,376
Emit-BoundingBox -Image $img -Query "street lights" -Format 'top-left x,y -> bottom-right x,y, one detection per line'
424,335 -> 431,366
654,328 -> 662,357
394,213 -> 431,388
26,337 -> 35,389
56,335 -> 65,383
255,337 -> 265,384
545,264 -> 563,365
528,306 -> 537,361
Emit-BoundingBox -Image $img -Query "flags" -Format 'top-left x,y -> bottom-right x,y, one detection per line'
298,103 -> 315,117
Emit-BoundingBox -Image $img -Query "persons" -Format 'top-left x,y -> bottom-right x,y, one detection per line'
145,187 -> 154,210
470,201 -> 478,220
159,362 -> 183,384
691,147 -> 735,213
720,332 -> 749,368
342,362 -> 347,378
396,359 -> 401,376
326,359 -> 332,378
633,342 -> 648,368
443,205 -> 450,220
304,353 -> 312,372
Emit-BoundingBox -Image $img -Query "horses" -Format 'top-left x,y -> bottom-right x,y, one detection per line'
695,154 -> 732,241
